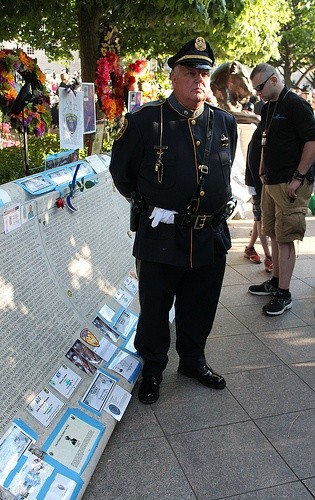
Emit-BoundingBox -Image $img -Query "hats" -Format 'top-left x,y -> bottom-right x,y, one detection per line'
167,36 -> 216,70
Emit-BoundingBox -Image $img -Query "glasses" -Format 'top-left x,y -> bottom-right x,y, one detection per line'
252,73 -> 275,91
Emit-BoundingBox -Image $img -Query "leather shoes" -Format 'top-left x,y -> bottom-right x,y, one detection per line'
138,373 -> 163,405
177,364 -> 227,390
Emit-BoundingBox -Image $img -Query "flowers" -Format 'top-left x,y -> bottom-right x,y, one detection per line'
96,50 -> 146,119
0,48 -> 55,135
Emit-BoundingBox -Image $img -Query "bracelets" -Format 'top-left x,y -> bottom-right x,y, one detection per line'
292,176 -> 303,182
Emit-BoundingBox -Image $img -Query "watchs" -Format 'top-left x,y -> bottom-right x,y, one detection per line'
292,170 -> 305,179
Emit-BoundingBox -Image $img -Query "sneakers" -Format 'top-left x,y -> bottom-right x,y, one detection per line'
244,244 -> 262,265
263,292 -> 293,315
263,255 -> 274,271
248,280 -> 278,295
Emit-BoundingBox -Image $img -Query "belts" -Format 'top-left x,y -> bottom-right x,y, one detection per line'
174,210 -> 218,229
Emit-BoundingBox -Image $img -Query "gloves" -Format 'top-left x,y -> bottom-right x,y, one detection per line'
149,206 -> 180,229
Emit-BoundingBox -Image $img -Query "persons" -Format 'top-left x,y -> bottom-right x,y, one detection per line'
107,35 -> 239,406
210,59 -> 315,117
249,63 -> 315,317
243,100 -> 274,273
56,73 -> 71,95
